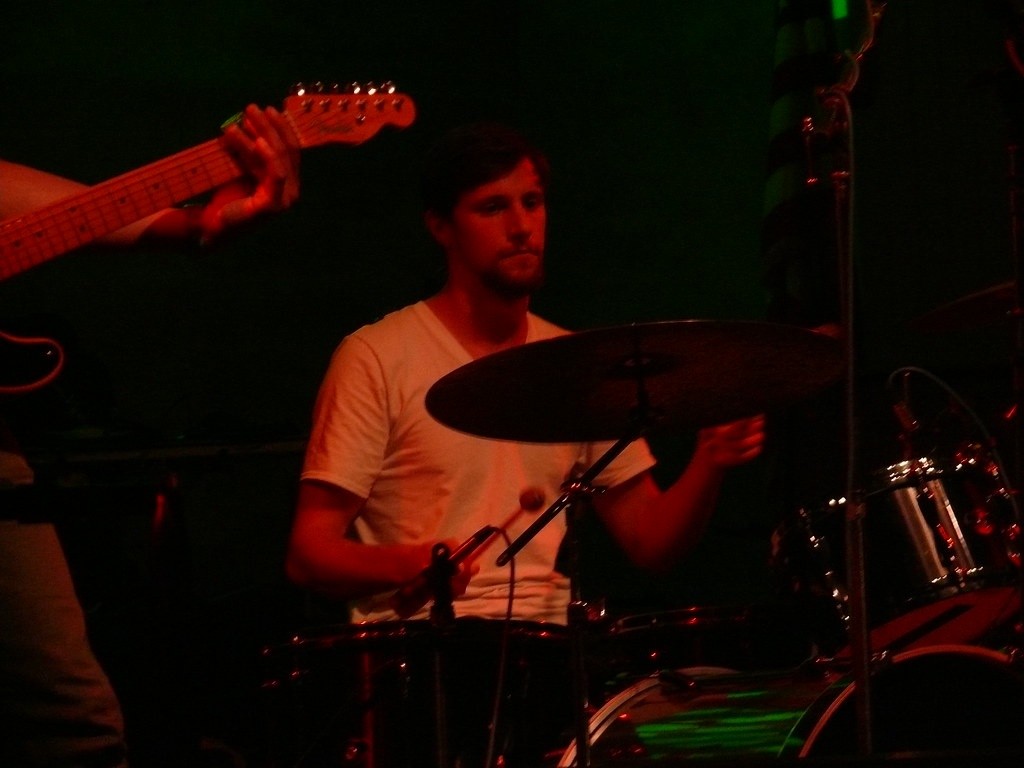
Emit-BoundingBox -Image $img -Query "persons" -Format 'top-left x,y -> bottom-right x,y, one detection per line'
284,121 -> 769,767
1,103 -> 301,768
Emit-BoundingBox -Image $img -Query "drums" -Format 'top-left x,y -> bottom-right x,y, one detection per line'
605,600 -> 753,675
781,444 -> 1024,655
280,615 -> 582,767
552,638 -> 1022,767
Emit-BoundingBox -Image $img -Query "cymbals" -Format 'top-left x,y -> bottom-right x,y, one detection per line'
427,305 -> 852,447
909,279 -> 1024,337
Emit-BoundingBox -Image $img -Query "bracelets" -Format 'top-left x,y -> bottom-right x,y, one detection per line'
180,204 -> 204,247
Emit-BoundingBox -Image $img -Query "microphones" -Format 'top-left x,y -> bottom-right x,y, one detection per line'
884,384 -> 920,437
392,524 -> 494,618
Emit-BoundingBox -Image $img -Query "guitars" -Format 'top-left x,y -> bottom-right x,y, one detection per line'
1,76 -> 419,401
